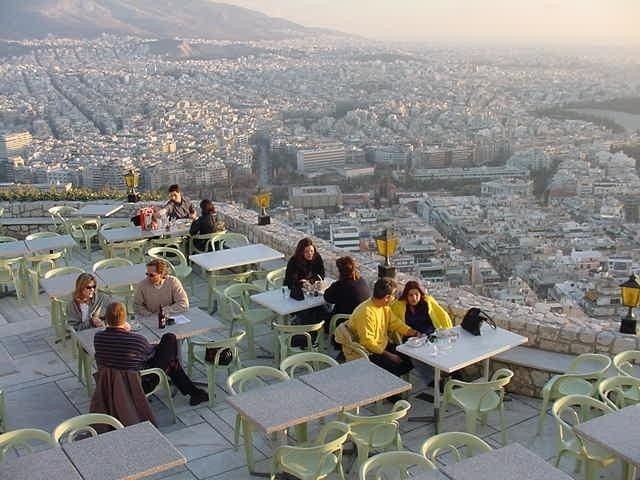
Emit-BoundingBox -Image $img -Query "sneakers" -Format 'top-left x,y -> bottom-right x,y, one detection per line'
189,390 -> 217,406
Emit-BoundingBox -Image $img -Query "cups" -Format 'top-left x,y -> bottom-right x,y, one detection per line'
281,281 -> 322,307
160,215 -> 186,232
166,319 -> 176,326
131,322 -> 142,331
408,329 -> 461,358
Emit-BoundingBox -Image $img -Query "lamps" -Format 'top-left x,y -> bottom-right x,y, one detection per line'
122,170 -> 143,202
249,187 -> 274,226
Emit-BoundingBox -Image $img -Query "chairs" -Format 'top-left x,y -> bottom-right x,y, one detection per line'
420,431 -> 493,468
613,350 -> 638,377
598,376 -> 640,409
67,327 -> 100,398
341,400 -> 412,468
271,320 -> 326,363
140,367 -> 177,426
0,428 -> 52,465
337,320 -> 410,395
536,351 -> 612,437
2,201 -> 284,341
358,450 -> 435,480
184,328 -> 248,408
273,421 -> 350,480
51,412 -> 123,448
437,368 -> 514,447
330,313 -> 352,339
225,365 -> 296,472
223,282 -> 275,360
279,351 -> 341,423
552,393 -> 620,479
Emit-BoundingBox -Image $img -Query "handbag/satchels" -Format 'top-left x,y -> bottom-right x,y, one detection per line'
205,340 -> 232,365
460,308 -> 496,335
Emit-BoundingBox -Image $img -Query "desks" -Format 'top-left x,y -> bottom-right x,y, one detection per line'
298,358 -> 413,430
60,421 -> 188,479
230,357 -> 342,475
572,403 -> 639,467
393,321 -> 529,431
439,442 -> 570,479
72,319 -> 160,395
2,447 -> 83,478
249,280 -> 326,327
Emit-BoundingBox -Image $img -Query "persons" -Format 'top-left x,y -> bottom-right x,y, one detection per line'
133,259 -> 190,318
155,184 -> 196,219
190,199 -> 224,252
283,238 -> 453,402
66,274 -> 113,331
95,302 -> 215,406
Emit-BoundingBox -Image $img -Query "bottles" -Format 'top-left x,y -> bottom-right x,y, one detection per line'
158,304 -> 165,329
